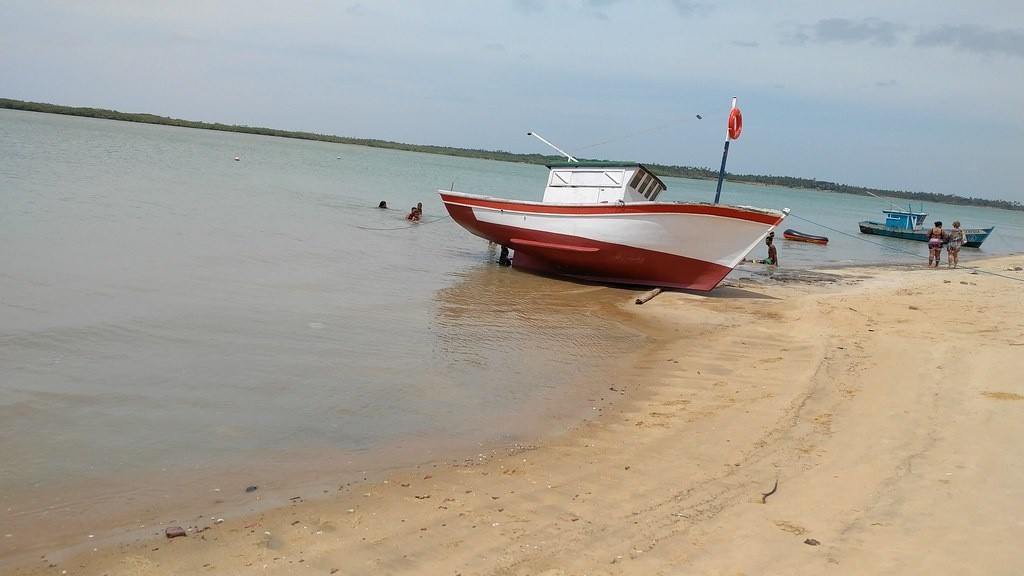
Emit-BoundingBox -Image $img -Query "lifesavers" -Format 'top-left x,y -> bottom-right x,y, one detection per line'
729,108 -> 743,140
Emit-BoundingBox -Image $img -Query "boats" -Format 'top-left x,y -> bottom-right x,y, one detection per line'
857,202 -> 995,249
436,95 -> 792,295
784,229 -> 828,246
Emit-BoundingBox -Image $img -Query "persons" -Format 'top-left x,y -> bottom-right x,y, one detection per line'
747,236 -> 780,266
926,221 -> 946,268
417,202 -> 422,214
769,232 -> 775,236
379,201 -> 387,208
405,207 -> 419,221
946,221 -> 964,269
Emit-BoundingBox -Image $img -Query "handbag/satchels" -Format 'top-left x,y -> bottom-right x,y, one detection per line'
961,229 -> 967,246
